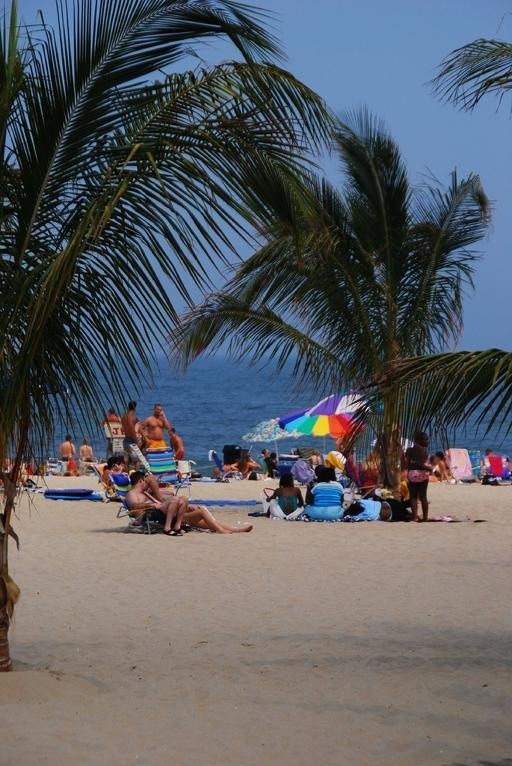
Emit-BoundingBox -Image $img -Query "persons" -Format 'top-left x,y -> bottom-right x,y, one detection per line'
0,457 -> 27,487
225,448 -> 322,485
59,435 -> 93,463
402,431 -> 433,523
428,451 -> 449,482
480,449 -> 504,477
101,456 -> 253,534
102,401 -> 184,471
264,465 -> 345,520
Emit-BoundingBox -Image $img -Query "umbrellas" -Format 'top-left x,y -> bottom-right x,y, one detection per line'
305,386 -> 389,469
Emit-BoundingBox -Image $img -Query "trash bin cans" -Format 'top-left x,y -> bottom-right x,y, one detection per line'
223,444 -> 241,464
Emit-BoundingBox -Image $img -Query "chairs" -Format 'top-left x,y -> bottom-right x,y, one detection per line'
208,450 -> 243,484
92,461 -> 200,537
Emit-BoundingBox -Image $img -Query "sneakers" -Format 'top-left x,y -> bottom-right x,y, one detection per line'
162,528 -> 183,536
